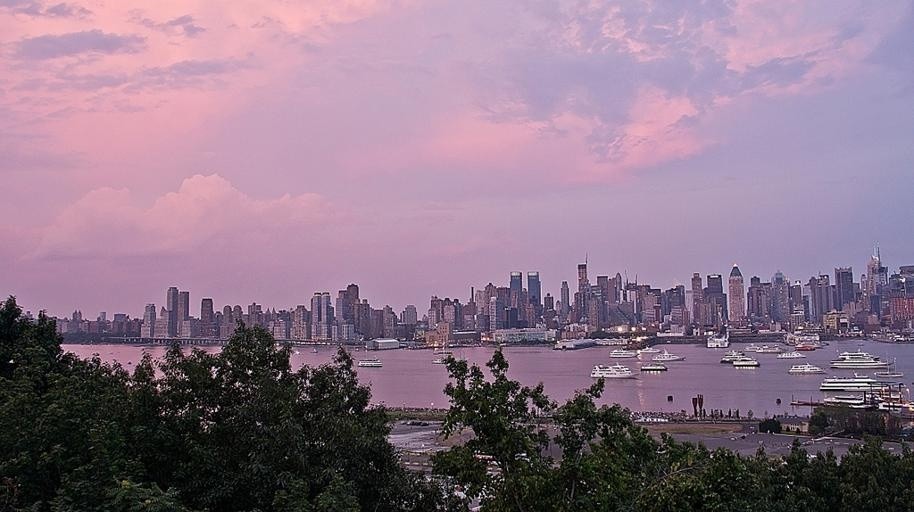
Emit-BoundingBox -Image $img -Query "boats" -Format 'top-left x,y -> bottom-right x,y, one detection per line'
358,356 -> 383,367
719,333 -> 832,377
590,343 -> 687,379
432,351 -> 454,364
815,343 -> 914,422
876,333 -> 914,342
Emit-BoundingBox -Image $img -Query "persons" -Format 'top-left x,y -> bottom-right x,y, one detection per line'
699,408 -> 740,420
